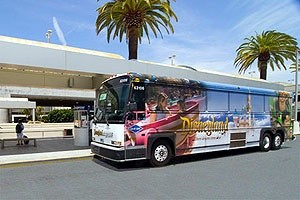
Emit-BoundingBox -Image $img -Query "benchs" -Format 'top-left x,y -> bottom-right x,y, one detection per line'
0,138 -> 38,149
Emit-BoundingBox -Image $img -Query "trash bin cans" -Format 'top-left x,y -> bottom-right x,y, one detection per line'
64,129 -> 72,138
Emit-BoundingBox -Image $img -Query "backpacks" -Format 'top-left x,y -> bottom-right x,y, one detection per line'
16,125 -> 23,134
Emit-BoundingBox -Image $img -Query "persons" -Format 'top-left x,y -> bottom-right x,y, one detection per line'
176,99 -> 186,115
15,118 -> 24,145
152,92 -> 173,123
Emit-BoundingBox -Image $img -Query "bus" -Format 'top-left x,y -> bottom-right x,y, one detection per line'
89,72 -> 294,168
